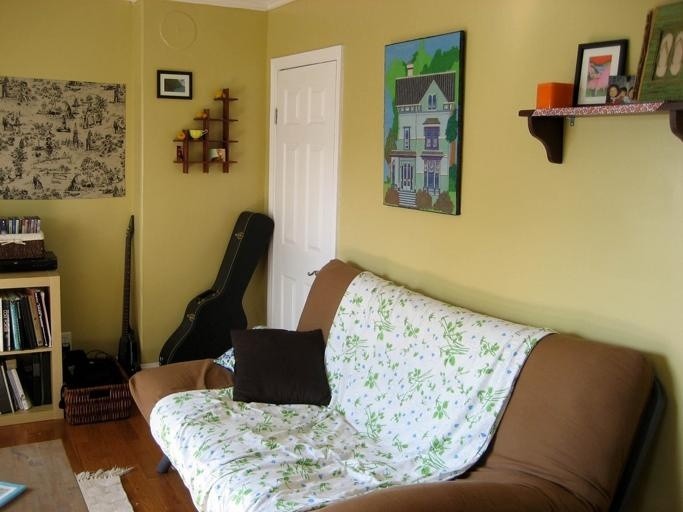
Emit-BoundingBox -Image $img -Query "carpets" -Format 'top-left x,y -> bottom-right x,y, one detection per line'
73,466 -> 137,512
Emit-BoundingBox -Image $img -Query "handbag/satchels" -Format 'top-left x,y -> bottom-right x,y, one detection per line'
64,350 -> 122,389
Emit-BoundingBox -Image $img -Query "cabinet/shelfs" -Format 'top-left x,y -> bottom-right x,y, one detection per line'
0,270 -> 64,447
173,88 -> 239,174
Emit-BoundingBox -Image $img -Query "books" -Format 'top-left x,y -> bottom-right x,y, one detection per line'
0,481 -> 27,508
0,217 -> 52,414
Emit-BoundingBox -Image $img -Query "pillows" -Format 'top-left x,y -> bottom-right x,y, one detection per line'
230,328 -> 332,405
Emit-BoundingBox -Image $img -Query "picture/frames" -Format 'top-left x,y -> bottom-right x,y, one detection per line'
572,38 -> 628,106
381,31 -> 465,216
157,69 -> 192,100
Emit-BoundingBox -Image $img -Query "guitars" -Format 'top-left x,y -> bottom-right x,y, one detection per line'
118,215 -> 140,377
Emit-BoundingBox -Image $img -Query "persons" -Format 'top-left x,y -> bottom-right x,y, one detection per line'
607,75 -> 636,104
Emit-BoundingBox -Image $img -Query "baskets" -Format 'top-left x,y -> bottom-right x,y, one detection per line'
64,357 -> 133,425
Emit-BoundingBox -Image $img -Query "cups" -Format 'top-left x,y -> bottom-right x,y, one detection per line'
189,129 -> 209,141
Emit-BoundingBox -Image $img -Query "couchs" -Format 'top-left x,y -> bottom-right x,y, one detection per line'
126,259 -> 669,511
